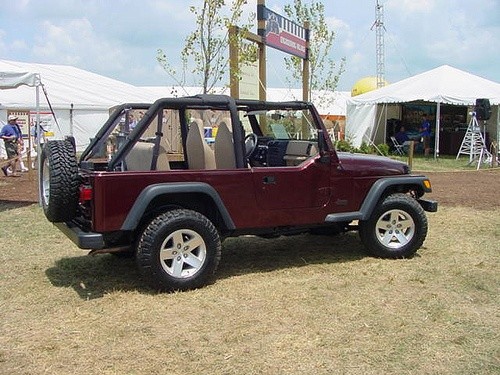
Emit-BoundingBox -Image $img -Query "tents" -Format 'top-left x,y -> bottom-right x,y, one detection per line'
138,84 -> 351,151
344,64 -> 500,161
0,59 -> 173,158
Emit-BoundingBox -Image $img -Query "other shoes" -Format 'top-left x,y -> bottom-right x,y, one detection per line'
11,173 -> 20,177
21,168 -> 29,173
7,169 -> 13,173
2,167 -> 8,177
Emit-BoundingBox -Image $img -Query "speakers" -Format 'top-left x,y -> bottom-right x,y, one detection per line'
475,99 -> 492,120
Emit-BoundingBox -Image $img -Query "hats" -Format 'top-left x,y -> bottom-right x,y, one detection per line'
34,120 -> 42,123
8,115 -> 18,121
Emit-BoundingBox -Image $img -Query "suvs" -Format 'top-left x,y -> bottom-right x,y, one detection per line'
38,93 -> 439,295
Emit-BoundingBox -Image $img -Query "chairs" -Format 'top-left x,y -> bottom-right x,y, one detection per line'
390,136 -> 405,157
186,118 -> 236,169
125,142 -> 170,171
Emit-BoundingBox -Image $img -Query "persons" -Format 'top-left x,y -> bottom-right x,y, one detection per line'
396,110 -> 432,155
0,115 -> 30,177
30,119 -> 48,169
